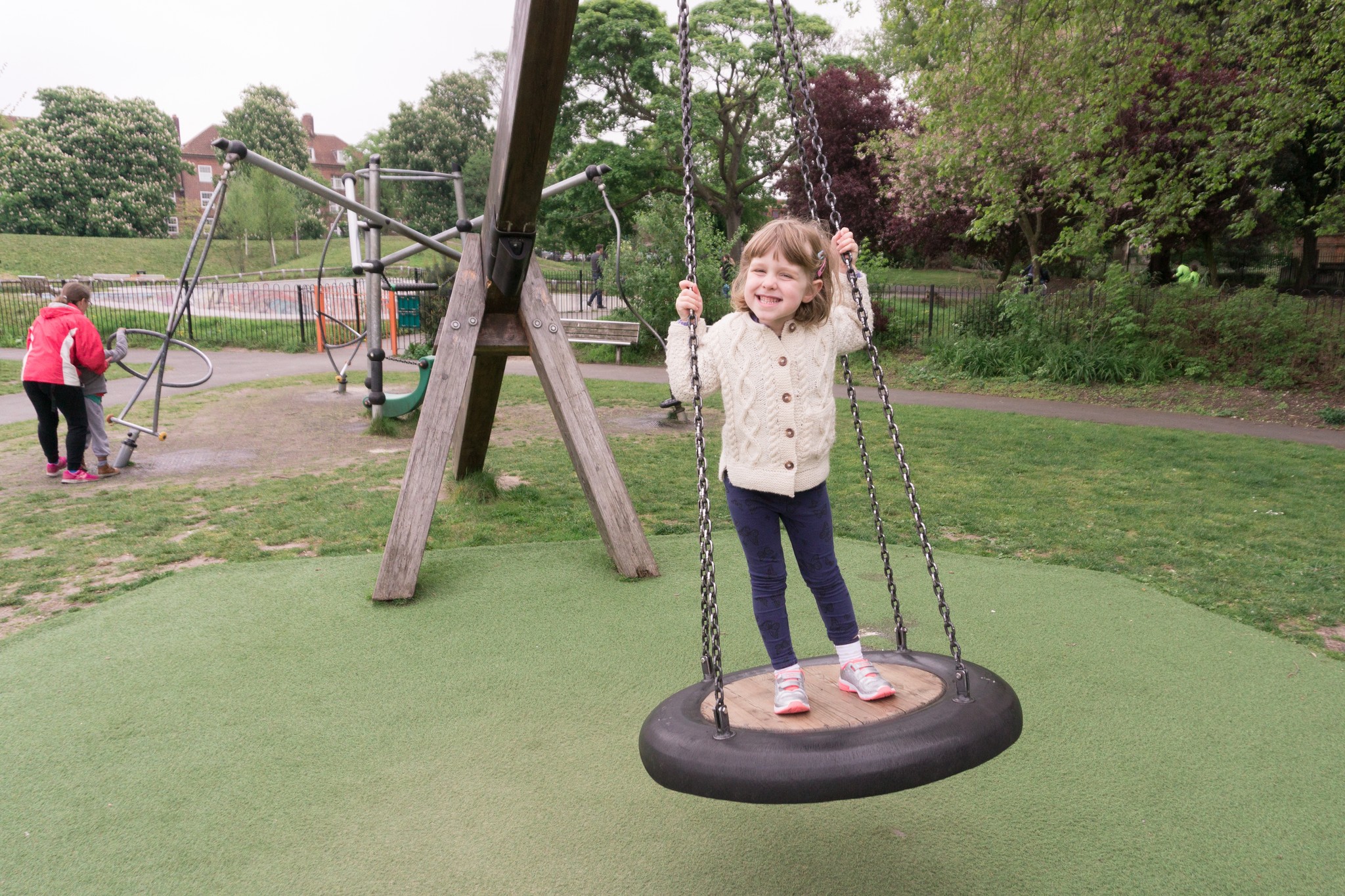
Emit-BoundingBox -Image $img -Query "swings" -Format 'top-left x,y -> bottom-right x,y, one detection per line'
635,0 -> 1025,805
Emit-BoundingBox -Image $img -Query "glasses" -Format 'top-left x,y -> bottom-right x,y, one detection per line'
80,298 -> 92,308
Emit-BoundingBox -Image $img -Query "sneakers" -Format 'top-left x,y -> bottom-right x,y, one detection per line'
97,463 -> 120,478
61,468 -> 99,483
47,456 -> 67,477
839,658 -> 895,700
80,455 -> 87,470
772,667 -> 811,715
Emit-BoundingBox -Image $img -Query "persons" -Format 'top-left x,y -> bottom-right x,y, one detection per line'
21,282 -> 113,484
80,327 -> 128,479
665,210 -> 895,714
587,244 -> 608,308
